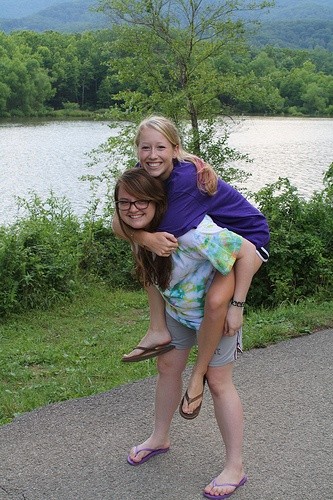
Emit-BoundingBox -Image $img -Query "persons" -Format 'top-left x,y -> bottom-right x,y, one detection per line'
113,169 -> 255,500
120,112 -> 270,420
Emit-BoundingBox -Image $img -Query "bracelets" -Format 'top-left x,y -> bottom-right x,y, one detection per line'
231,301 -> 246,307
131,229 -> 136,237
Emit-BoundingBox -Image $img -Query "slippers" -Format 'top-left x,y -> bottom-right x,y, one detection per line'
180,374 -> 208,420
203,473 -> 248,500
121,345 -> 176,365
127,443 -> 170,466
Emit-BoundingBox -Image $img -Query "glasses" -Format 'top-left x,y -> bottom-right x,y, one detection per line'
115,199 -> 152,211
